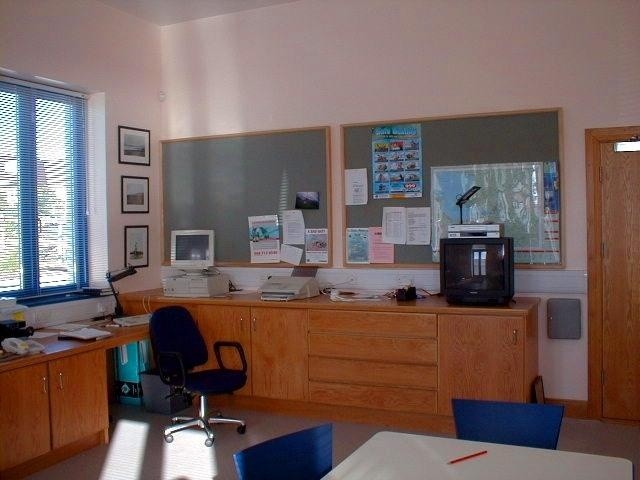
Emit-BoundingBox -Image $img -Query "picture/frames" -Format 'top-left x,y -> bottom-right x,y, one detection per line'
122,176 -> 149,213
118,126 -> 150,166
125,226 -> 149,268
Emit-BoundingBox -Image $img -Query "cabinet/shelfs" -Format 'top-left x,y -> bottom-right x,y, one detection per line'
0,315 -> 150,480
117,288 -> 541,435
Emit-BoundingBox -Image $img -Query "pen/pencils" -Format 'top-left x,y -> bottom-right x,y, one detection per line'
448,450 -> 487,463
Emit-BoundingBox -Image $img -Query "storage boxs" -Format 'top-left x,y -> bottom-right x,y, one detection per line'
112,339 -> 151,407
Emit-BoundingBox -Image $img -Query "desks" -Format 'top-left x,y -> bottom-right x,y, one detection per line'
319,431 -> 633,480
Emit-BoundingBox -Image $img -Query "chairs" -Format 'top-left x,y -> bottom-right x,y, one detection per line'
233,423 -> 332,480
150,306 -> 246,447
452,400 -> 564,450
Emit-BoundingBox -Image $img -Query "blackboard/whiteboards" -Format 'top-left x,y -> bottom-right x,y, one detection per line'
160,125 -> 334,269
339,106 -> 564,269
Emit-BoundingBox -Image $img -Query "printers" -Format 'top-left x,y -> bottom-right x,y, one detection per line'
257,265 -> 321,302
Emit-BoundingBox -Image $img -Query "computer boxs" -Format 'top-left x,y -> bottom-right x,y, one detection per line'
160,276 -> 231,297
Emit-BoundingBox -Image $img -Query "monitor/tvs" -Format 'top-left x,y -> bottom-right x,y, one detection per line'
171,229 -> 215,276
439,237 -> 514,307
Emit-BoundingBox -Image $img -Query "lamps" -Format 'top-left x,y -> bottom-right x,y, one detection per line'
105,266 -> 137,321
456,185 -> 481,225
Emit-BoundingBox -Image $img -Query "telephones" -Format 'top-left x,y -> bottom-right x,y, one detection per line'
1,338 -> 44,355
